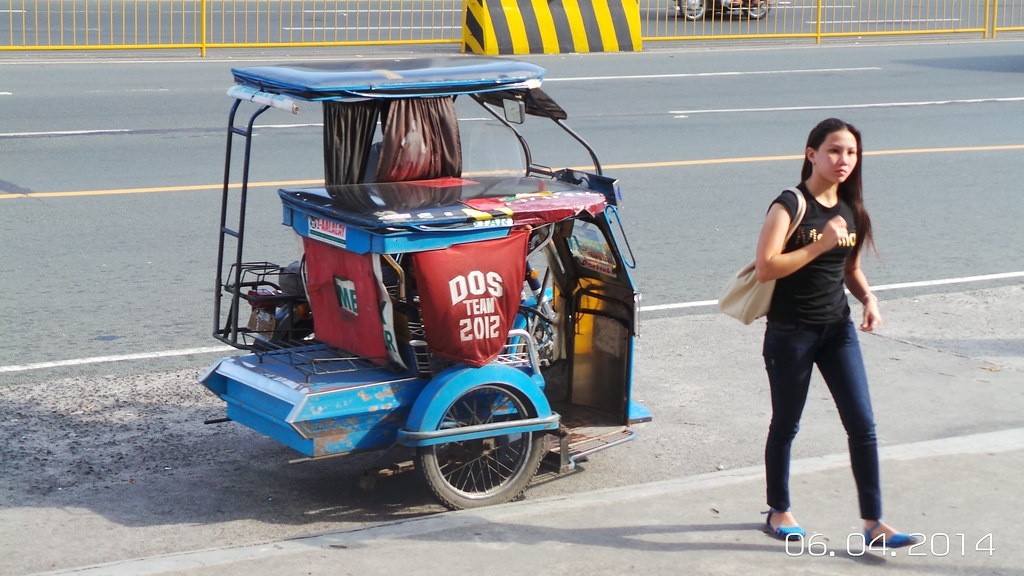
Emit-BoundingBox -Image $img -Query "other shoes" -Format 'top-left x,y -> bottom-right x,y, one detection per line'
863,521 -> 917,548
761,508 -> 806,541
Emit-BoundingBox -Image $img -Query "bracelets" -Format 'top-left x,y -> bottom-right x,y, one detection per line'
861,293 -> 878,305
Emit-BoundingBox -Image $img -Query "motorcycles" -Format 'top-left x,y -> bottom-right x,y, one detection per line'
675,0 -> 773,20
197,59 -> 655,508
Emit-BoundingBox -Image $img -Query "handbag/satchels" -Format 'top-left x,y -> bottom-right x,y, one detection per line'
718,187 -> 807,325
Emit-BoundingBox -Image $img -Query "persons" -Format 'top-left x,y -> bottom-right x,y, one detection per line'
363,99 -> 392,184
757,116 -> 918,548
